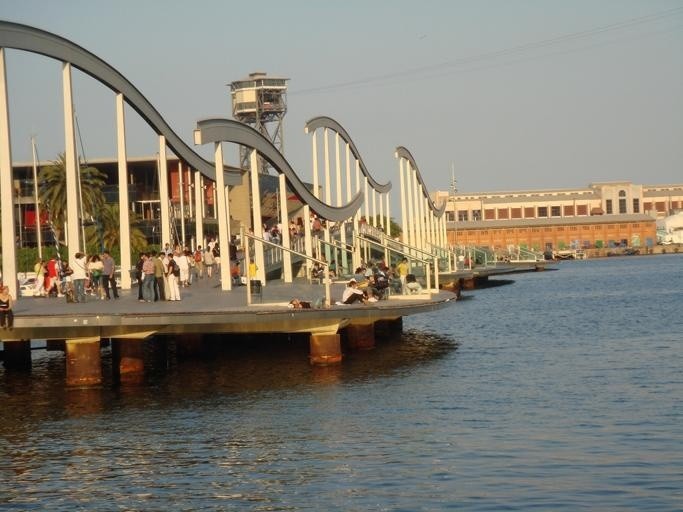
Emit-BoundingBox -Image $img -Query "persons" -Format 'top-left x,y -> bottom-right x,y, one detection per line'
464,257 -> 469,266
0,286 -> 13,329
458,254 -> 464,265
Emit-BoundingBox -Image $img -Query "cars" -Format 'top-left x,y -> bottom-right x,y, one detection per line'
19,277 -> 35,285
19,284 -> 34,296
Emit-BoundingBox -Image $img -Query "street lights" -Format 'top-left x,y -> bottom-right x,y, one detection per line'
448,176 -> 459,247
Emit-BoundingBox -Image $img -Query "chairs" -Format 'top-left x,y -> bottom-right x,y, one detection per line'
308,268 -> 321,285
